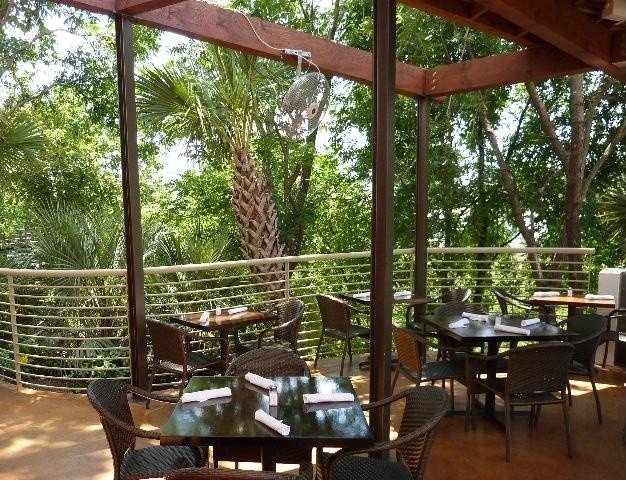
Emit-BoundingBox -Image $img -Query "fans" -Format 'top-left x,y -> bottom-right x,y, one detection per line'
278,49 -> 330,141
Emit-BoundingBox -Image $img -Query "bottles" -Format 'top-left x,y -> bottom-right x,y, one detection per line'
269,406 -> 277,419
216,316 -> 222,325
269,386 -> 278,406
216,305 -> 222,316
495,313 -> 501,325
567,287 -> 572,297
